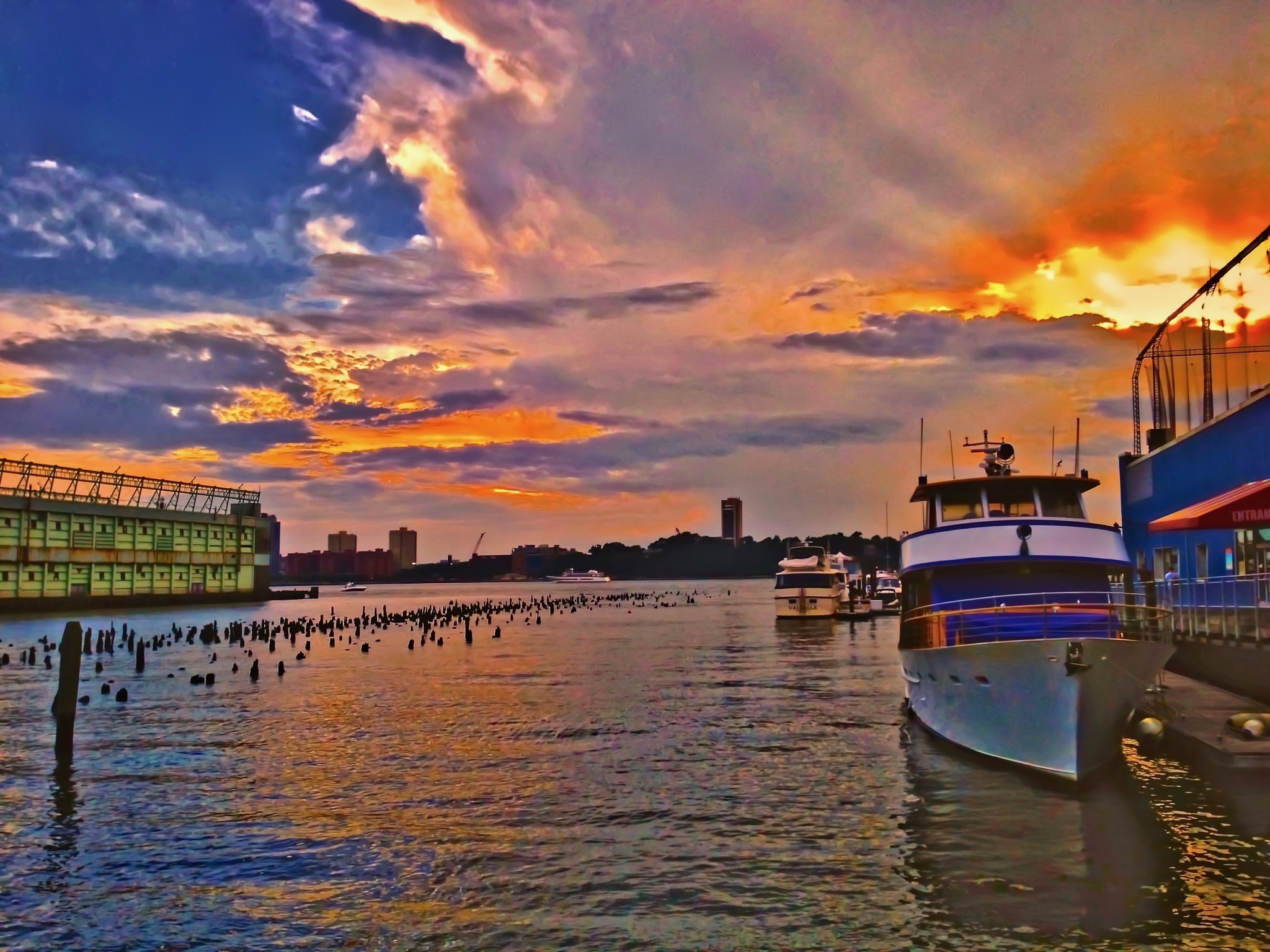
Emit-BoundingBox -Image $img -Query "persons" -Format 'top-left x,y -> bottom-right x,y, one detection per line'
1137,564 -> 1157,627
1165,567 -> 1180,579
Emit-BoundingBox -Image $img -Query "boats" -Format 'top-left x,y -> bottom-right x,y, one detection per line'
773,538 -> 901,620
340,581 -> 368,592
546,568 -> 610,584
897,413 -> 1181,785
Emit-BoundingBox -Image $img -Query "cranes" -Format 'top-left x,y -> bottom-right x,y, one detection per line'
471,532 -> 486,557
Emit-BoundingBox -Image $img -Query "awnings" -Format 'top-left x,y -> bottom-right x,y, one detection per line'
1147,478 -> 1270,530
